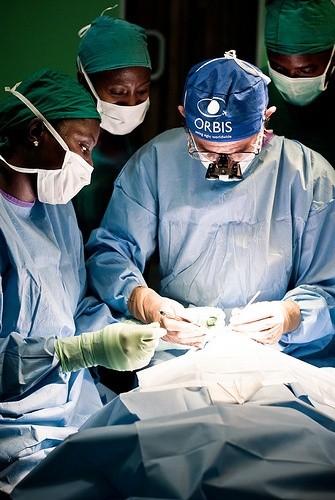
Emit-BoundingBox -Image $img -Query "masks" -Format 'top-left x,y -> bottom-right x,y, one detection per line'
268,42 -> 335,108
85,71 -> 150,136
37,117 -> 94,205
184,125 -> 266,181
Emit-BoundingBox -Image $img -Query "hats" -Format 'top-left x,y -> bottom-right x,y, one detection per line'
182,58 -> 269,142
0,69 -> 102,150
77,15 -> 153,80
263,0 -> 335,55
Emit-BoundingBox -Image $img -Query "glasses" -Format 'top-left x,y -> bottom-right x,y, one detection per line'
183,121 -> 262,162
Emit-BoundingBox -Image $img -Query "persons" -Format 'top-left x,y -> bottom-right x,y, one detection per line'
68,15 -> 161,293
258,0 -> 335,171
0,68 -> 168,500
86,54 -> 335,384
